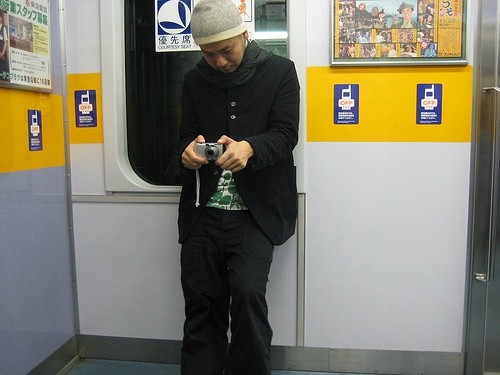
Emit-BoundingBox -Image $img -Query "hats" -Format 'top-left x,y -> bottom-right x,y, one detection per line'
191,0 -> 249,45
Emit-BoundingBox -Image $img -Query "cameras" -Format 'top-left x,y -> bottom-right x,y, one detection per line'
195,142 -> 223,160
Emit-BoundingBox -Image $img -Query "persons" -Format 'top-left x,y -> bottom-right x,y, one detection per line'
336,0 -> 436,62
0,10 -> 9,75
161,0 -> 300,375
